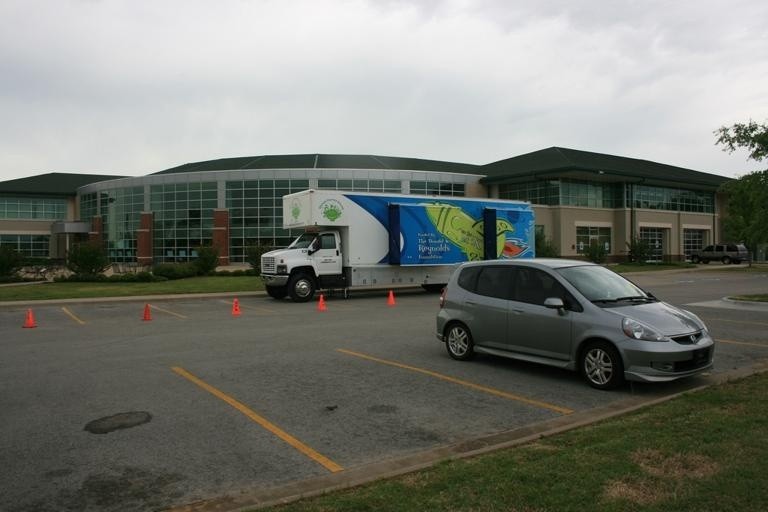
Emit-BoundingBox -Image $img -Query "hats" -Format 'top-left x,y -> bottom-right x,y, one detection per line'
434,257 -> 719,391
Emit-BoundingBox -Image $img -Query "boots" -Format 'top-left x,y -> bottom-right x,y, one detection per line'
690,243 -> 748,266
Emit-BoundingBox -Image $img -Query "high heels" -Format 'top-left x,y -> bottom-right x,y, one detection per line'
140,302 -> 153,321
317,294 -> 328,311
21,307 -> 37,328
386,290 -> 396,306
231,297 -> 241,315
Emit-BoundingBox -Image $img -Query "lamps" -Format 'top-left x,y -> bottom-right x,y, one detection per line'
523,277 -> 546,305
553,279 -> 569,304
